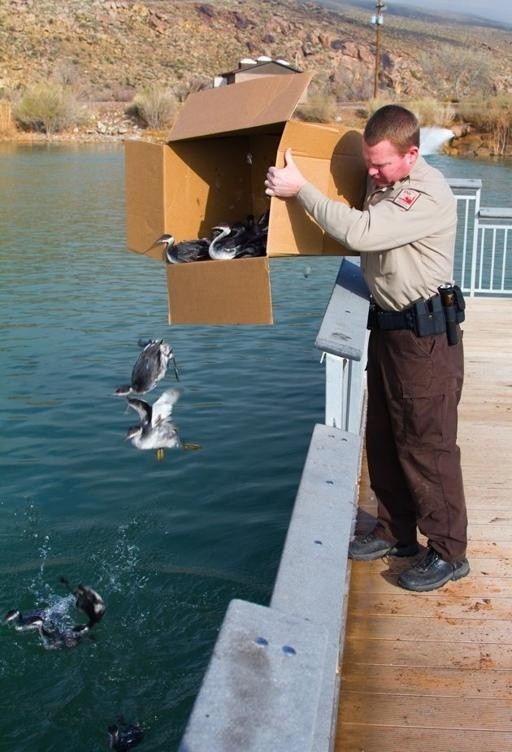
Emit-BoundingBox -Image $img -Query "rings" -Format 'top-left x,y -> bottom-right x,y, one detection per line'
269,175 -> 273,182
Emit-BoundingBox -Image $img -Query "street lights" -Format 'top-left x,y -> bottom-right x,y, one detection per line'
372,1 -> 387,101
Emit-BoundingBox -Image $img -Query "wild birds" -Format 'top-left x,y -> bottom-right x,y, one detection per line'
2,575 -> 108,651
113,337 -> 180,396
106,714 -> 144,752
122,387 -> 203,463
154,207 -> 270,264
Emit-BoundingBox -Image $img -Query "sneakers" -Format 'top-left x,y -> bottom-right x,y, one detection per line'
398,547 -> 470,592
348,532 -> 420,558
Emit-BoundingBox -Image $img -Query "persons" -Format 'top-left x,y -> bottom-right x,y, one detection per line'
266,104 -> 471,592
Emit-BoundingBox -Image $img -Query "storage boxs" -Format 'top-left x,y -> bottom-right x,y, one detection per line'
121,70 -> 372,330
